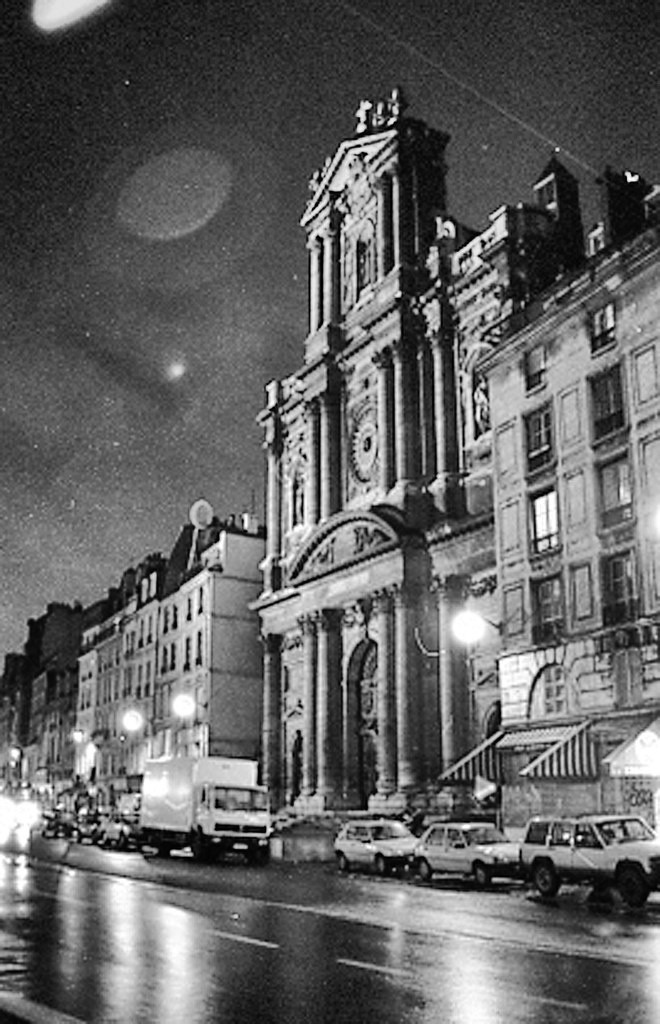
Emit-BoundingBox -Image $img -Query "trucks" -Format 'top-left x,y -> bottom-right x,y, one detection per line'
138,758 -> 271,863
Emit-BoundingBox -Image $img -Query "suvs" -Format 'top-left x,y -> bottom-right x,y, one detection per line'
516,815 -> 660,906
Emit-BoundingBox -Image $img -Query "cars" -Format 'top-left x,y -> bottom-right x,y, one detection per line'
71,816 -> 139,851
414,821 -> 520,885
333,820 -> 420,876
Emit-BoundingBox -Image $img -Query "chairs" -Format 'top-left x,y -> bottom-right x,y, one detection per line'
605,828 -> 615,838
